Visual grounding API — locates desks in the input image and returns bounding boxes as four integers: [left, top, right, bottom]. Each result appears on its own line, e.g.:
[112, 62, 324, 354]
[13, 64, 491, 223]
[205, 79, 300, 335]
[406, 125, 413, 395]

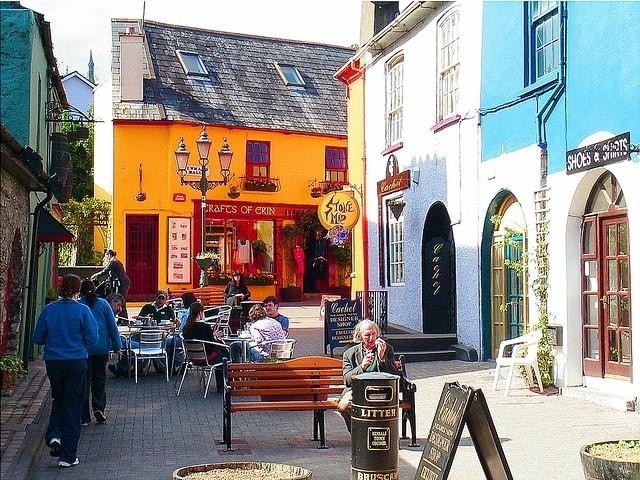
[129, 323, 176, 375]
[222, 336, 266, 362]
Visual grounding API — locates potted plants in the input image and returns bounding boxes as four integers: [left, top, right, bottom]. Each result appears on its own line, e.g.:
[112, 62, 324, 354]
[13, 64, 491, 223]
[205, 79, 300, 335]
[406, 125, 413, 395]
[580, 440, 640, 480]
[0, 354, 27, 392]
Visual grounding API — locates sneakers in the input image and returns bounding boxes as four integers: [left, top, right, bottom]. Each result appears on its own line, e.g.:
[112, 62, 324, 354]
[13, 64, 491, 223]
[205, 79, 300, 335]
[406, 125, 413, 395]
[108, 363, 121, 378]
[123, 368, 135, 377]
[59, 458, 79, 467]
[94, 410, 107, 424]
[50, 438, 61, 457]
[81, 423, 90, 427]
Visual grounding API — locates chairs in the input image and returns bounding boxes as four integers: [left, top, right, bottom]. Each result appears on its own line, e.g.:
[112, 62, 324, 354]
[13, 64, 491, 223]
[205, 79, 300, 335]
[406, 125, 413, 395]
[129, 329, 169, 383]
[200, 314, 224, 332]
[167, 298, 201, 311]
[493, 329, 544, 396]
[204, 305, 232, 335]
[246, 339, 296, 362]
[177, 339, 236, 399]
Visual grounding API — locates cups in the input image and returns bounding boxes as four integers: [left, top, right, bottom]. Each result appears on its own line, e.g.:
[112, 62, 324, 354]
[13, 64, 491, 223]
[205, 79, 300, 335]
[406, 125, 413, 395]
[240, 331, 248, 337]
[224, 327, 228, 337]
[160, 320, 169, 326]
[135, 320, 142, 326]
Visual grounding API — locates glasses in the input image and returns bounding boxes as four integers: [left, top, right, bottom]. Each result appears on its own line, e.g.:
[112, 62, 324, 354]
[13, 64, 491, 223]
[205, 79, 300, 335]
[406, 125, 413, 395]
[158, 298, 165, 300]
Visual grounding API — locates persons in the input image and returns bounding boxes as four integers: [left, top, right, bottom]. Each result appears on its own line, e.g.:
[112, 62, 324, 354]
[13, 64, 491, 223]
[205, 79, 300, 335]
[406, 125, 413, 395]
[82, 279, 123, 427]
[224, 271, 251, 302]
[263, 296, 290, 331]
[183, 302, 230, 394]
[166, 292, 198, 376]
[231, 303, 286, 362]
[32, 274, 99, 466]
[337, 320, 404, 435]
[138, 292, 175, 323]
[91, 249, 132, 325]
[105, 292, 139, 377]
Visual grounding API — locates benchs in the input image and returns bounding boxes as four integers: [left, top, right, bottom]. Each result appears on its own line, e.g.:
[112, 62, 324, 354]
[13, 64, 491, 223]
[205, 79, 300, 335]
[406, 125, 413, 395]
[222, 355, 420, 452]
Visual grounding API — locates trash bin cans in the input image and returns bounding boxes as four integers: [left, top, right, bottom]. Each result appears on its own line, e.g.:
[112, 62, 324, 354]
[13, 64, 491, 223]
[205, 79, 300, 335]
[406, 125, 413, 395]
[352, 372, 399, 480]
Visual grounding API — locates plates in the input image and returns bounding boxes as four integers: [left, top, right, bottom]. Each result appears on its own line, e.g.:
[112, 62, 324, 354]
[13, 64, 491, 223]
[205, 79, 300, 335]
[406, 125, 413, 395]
[159, 322, 174, 327]
[132, 323, 144, 325]
[239, 336, 251, 338]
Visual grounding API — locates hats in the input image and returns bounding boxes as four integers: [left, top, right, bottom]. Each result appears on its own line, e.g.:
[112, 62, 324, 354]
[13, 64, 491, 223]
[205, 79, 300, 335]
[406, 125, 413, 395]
[157, 290, 167, 298]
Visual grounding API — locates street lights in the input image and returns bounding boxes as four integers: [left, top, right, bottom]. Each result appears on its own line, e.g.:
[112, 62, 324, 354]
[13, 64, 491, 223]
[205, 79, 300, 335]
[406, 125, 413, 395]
[173, 122, 236, 288]
